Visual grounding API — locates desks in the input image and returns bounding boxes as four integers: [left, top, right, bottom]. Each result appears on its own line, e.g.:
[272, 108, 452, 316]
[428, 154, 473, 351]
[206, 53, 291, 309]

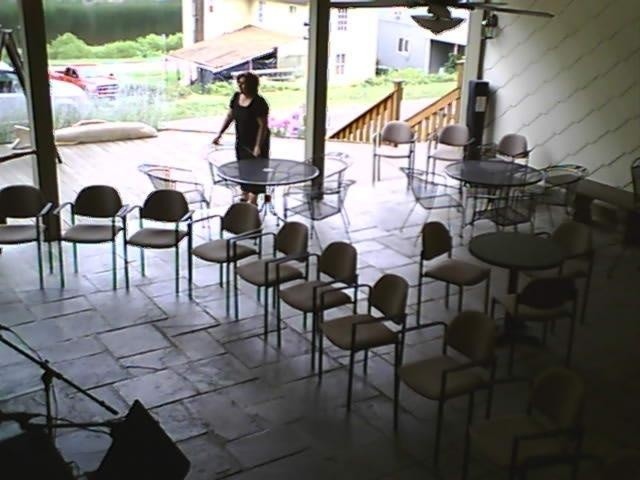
[216, 159, 320, 246]
[469, 232, 570, 347]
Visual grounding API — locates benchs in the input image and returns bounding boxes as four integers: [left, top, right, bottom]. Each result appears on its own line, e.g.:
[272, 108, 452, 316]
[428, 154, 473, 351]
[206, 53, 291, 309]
[546, 171, 639, 246]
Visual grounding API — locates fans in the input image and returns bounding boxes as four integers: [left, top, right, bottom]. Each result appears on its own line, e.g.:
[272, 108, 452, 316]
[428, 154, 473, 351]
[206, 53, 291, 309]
[328, 0, 559, 36]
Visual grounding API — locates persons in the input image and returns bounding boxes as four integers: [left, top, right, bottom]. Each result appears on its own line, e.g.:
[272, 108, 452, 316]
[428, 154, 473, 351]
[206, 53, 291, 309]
[212, 71, 272, 207]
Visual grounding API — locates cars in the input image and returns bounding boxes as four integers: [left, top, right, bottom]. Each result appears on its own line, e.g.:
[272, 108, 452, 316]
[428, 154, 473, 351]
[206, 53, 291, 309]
[0, 61, 89, 127]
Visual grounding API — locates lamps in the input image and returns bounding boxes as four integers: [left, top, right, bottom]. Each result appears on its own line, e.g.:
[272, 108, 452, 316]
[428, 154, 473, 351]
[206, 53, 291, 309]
[408, 3, 465, 36]
[479, 11, 501, 39]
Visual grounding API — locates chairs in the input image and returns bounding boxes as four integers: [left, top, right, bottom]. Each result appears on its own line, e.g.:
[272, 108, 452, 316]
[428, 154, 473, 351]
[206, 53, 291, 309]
[529, 220, 594, 344]
[486, 275, 580, 374]
[122, 188, 194, 297]
[1, 184, 54, 289]
[462, 366, 588, 477]
[372, 121, 417, 191]
[394, 310, 497, 461]
[605, 158, 639, 284]
[416, 221, 492, 324]
[203, 147, 276, 213]
[317, 273, 410, 414]
[275, 241, 359, 374]
[137, 164, 215, 241]
[398, 134, 589, 247]
[424, 124, 475, 192]
[53, 185, 130, 293]
[231, 222, 309, 346]
[190, 202, 263, 315]
[282, 151, 356, 255]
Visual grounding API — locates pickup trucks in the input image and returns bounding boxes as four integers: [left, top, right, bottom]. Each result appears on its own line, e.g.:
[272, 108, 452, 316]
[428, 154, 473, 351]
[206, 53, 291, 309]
[50, 63, 120, 101]
